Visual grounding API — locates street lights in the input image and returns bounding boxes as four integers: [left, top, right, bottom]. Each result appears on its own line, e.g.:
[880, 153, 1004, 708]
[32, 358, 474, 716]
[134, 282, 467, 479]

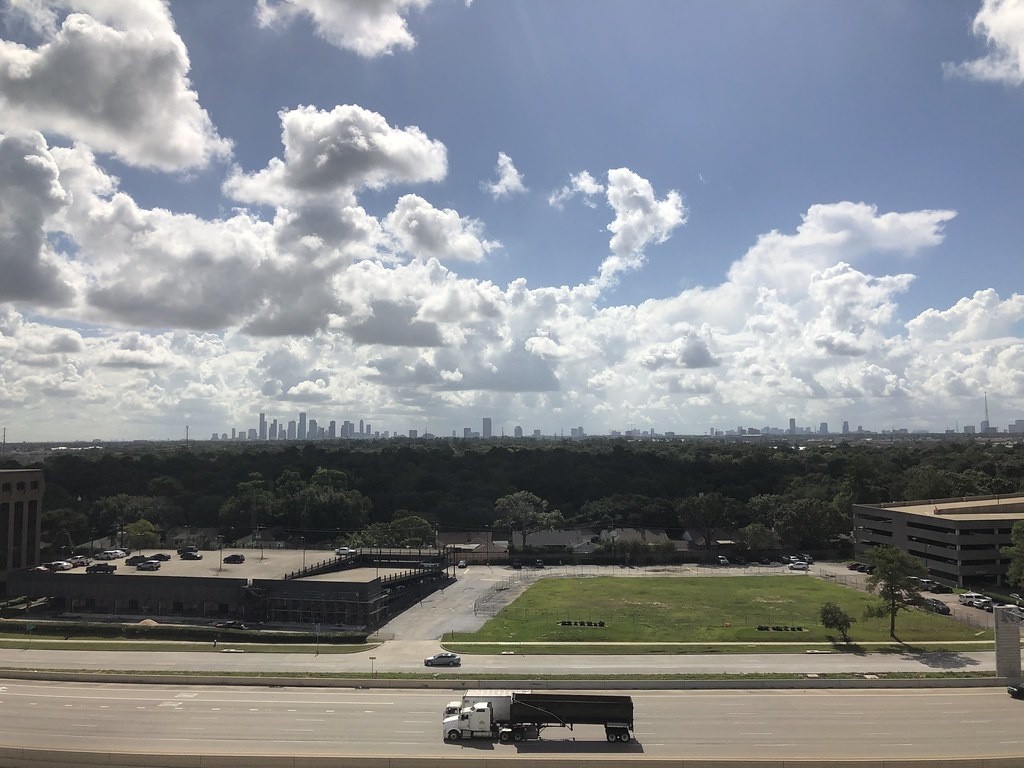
[185, 524, 191, 545]
[218, 534, 225, 570]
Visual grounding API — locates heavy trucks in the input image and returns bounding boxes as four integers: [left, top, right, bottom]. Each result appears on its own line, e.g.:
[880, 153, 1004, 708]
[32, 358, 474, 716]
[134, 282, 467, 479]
[442, 701, 540, 743]
[443, 689, 635, 741]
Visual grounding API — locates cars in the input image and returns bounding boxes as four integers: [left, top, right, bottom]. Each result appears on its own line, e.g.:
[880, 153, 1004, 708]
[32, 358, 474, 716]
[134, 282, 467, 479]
[1007, 681, 1024, 700]
[959, 593, 1005, 612]
[780, 554, 813, 570]
[717, 555, 729, 565]
[223, 554, 245, 563]
[29, 546, 203, 573]
[424, 653, 460, 666]
[216, 621, 249, 630]
[335, 547, 357, 555]
[847, 562, 953, 614]
[513, 558, 522, 568]
[458, 560, 468, 568]
[535, 559, 544, 568]
